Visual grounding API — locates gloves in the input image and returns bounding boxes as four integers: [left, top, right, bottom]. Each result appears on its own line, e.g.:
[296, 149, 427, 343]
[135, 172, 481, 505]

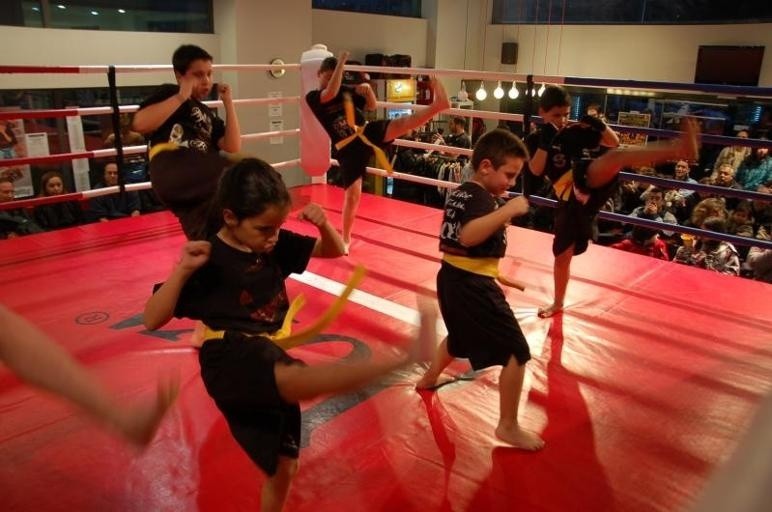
[577, 103, 608, 134]
[537, 122, 560, 151]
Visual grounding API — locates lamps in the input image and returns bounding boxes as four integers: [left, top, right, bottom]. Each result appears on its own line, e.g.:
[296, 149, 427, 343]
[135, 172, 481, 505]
[494, 0, 505, 100]
[477, 0, 486, 102]
[508, 0, 524, 99]
[538, 0, 553, 97]
[525, 0, 536, 97]
[457, 0, 471, 102]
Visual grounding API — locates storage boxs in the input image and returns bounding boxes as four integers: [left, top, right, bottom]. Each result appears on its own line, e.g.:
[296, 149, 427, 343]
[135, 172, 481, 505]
[365, 54, 411, 78]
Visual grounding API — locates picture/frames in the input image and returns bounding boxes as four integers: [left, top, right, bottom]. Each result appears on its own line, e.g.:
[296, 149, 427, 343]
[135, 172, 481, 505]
[617, 112, 651, 148]
[270, 58, 286, 78]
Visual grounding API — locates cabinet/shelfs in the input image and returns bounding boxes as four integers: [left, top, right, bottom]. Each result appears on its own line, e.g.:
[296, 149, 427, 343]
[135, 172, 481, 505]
[361, 78, 417, 195]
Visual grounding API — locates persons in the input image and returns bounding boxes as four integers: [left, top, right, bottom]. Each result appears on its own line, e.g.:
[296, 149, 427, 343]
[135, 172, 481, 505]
[597, 198, 625, 245]
[304, 50, 451, 255]
[417, 129, 546, 452]
[610, 221, 669, 261]
[671, 161, 700, 220]
[674, 217, 740, 276]
[745, 207, 772, 275]
[393, 130, 431, 201]
[618, 167, 644, 214]
[726, 201, 754, 239]
[0, 177, 43, 239]
[105, 114, 145, 159]
[744, 180, 772, 195]
[93, 163, 141, 220]
[677, 199, 724, 238]
[524, 84, 699, 318]
[432, 113, 470, 163]
[634, 167, 656, 195]
[736, 136, 772, 193]
[134, 45, 243, 243]
[1, 121, 17, 150]
[33, 172, 86, 232]
[145, 157, 438, 508]
[709, 162, 744, 191]
[621, 188, 678, 240]
[0, 303, 179, 453]
[711, 130, 751, 178]
